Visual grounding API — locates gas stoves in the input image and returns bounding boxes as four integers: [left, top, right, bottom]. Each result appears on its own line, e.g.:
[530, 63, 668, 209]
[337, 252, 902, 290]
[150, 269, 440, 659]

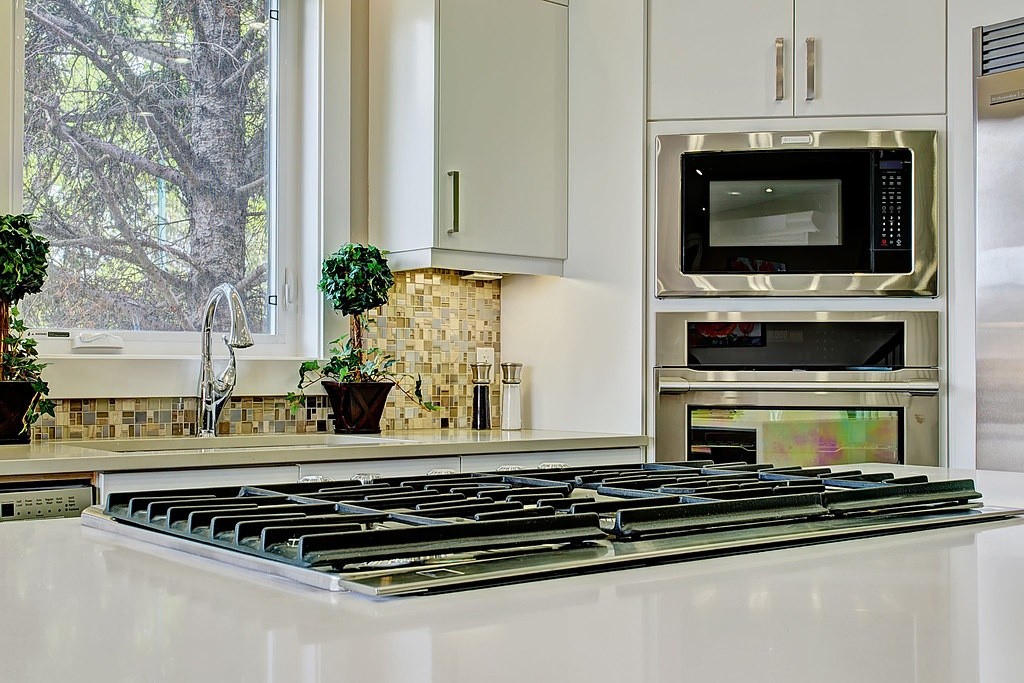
[81, 461, 1024, 595]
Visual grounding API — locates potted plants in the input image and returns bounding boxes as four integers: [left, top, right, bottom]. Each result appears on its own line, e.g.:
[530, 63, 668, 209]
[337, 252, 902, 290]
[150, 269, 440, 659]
[0, 212, 56, 445]
[282, 241, 441, 434]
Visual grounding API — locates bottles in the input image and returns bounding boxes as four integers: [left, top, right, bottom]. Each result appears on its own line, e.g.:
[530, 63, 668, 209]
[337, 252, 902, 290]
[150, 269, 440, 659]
[469, 363, 492, 430]
[501, 362, 522, 432]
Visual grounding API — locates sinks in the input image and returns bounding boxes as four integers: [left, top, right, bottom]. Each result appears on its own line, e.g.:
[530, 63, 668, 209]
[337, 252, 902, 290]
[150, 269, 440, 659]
[49, 434, 417, 452]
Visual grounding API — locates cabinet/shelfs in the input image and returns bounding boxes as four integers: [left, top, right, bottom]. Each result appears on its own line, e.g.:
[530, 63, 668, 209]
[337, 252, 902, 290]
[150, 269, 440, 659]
[369, 0, 571, 279]
[646, 0, 948, 121]
[99, 448, 641, 505]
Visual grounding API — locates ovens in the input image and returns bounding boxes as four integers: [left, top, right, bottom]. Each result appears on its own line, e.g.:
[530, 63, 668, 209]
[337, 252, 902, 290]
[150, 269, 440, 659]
[654, 312, 939, 466]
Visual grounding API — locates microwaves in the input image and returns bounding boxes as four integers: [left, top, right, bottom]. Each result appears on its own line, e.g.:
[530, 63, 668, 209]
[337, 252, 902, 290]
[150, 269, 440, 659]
[654, 130, 940, 298]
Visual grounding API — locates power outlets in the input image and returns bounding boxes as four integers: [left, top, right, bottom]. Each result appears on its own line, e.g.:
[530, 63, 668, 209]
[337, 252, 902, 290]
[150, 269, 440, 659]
[477, 346, 495, 385]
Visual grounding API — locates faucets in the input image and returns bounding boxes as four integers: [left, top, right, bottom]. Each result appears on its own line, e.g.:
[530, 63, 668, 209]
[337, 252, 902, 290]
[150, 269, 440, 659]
[198, 281, 255, 437]
